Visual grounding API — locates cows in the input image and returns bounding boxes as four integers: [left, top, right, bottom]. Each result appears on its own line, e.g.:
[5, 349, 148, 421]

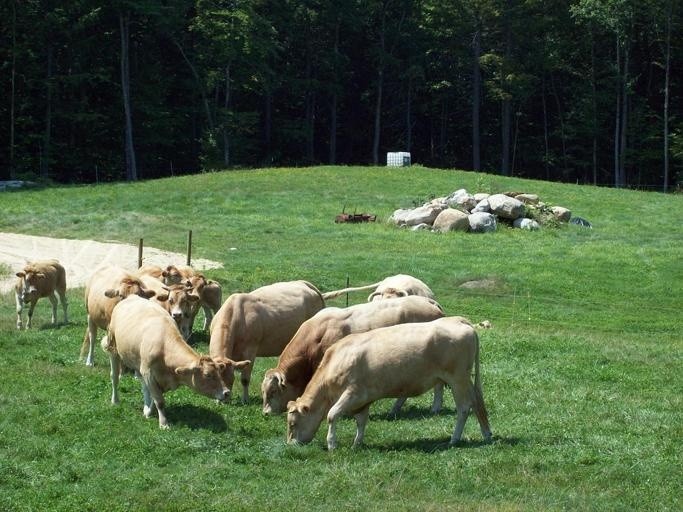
[205, 279, 326, 405]
[14, 260, 68, 331]
[260, 295, 446, 419]
[286, 316, 493, 453]
[105, 294, 231, 430]
[79, 263, 222, 379]
[321, 273, 435, 302]
